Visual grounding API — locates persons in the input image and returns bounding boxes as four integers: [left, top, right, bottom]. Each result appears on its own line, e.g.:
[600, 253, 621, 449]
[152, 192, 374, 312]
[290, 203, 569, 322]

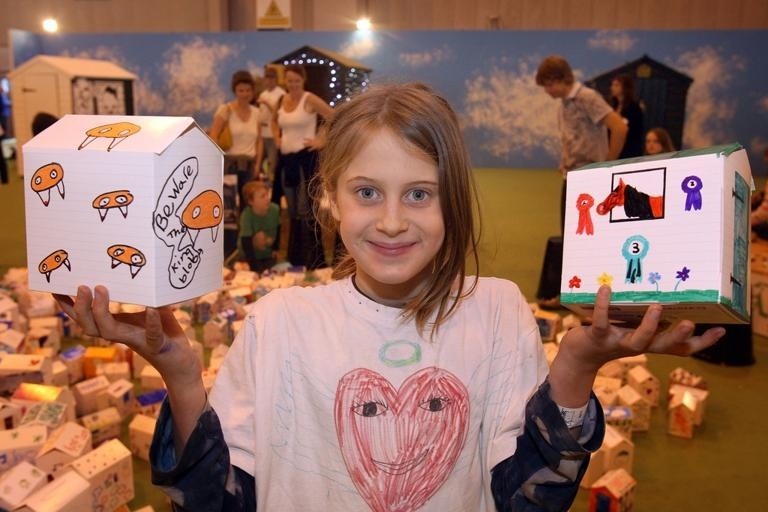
[48, 82, 726, 512]
[535, 57, 630, 312]
[210, 69, 264, 272]
[270, 62, 334, 271]
[643, 127, 676, 158]
[240, 182, 280, 273]
[255, 64, 286, 175]
[750, 180, 768, 246]
[601, 74, 647, 159]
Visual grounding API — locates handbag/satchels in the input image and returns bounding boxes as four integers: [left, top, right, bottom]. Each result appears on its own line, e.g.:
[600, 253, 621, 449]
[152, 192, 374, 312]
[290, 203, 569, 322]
[218, 103, 234, 152]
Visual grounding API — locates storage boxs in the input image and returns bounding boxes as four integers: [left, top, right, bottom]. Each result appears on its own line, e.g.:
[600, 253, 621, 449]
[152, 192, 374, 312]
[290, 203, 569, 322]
[19, 112, 230, 311]
[555, 137, 753, 330]
[3, 250, 746, 512]
